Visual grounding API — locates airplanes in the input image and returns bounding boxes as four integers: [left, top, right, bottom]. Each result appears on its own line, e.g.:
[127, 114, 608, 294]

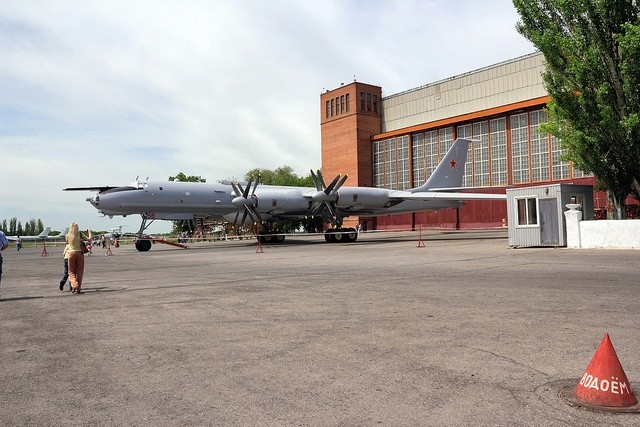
[5, 226, 69, 240]
[63, 137, 507, 252]
[92, 226, 122, 239]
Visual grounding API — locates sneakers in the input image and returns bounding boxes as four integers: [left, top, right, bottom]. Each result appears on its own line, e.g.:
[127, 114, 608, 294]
[75, 287, 80, 294]
[59, 281, 64, 290]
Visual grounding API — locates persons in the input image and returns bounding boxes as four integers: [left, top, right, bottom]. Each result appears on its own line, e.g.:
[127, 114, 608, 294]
[16, 235, 22, 250]
[101, 235, 106, 248]
[0, 231, 9, 283]
[59, 244, 72, 291]
[65, 223, 92, 294]
[176, 230, 236, 243]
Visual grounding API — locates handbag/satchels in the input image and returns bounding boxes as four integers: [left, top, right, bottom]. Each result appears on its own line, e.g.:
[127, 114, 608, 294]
[80, 233, 90, 254]
